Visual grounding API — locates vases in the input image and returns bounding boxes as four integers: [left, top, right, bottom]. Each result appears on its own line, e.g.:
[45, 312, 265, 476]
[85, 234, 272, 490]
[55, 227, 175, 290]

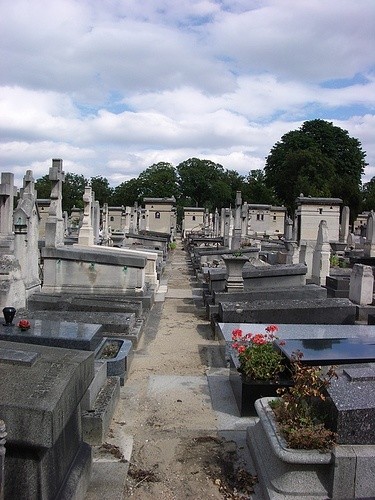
[229, 354, 296, 417]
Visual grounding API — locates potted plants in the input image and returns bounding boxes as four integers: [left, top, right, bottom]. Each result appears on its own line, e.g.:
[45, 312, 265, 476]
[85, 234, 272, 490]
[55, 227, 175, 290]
[245, 349, 340, 500]
[169, 243, 177, 253]
[93, 336, 134, 386]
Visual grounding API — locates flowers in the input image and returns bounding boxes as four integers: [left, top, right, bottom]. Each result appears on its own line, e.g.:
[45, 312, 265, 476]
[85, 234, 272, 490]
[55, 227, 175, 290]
[17, 320, 31, 332]
[232, 324, 286, 378]
